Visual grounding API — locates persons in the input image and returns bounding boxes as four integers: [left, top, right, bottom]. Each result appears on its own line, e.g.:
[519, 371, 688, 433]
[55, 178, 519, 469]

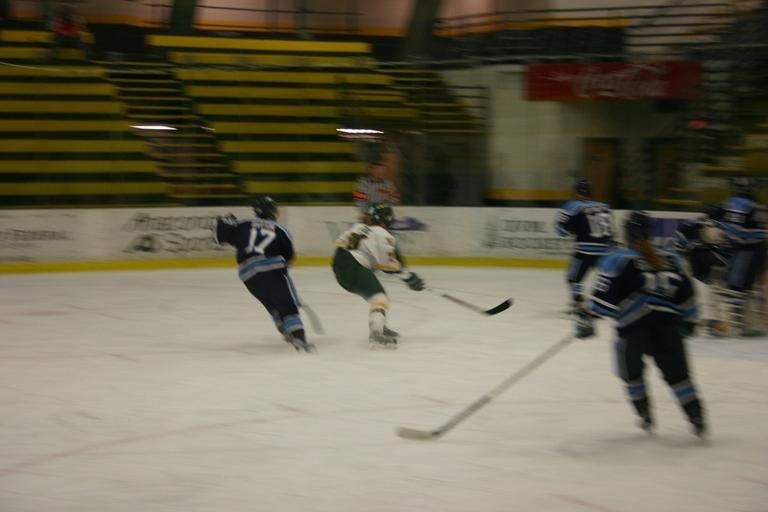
[353, 162, 400, 225]
[44, 4, 102, 66]
[554, 174, 768, 339]
[590, 209, 711, 437]
[330, 204, 423, 345]
[210, 195, 309, 352]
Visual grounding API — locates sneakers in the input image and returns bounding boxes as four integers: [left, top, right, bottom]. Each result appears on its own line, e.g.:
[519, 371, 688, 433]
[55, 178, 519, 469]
[368, 326, 401, 343]
[291, 337, 316, 351]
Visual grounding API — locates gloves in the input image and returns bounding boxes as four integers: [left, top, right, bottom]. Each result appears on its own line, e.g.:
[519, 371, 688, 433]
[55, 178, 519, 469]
[403, 272, 425, 291]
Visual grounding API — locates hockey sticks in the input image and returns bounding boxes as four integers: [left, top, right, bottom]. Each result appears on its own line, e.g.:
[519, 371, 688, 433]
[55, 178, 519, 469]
[419, 287, 513, 316]
[396, 334, 578, 439]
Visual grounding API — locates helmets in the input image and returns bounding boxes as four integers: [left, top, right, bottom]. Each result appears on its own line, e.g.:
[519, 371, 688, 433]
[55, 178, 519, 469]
[252, 194, 278, 220]
[367, 202, 395, 231]
[573, 178, 595, 197]
[625, 212, 651, 243]
